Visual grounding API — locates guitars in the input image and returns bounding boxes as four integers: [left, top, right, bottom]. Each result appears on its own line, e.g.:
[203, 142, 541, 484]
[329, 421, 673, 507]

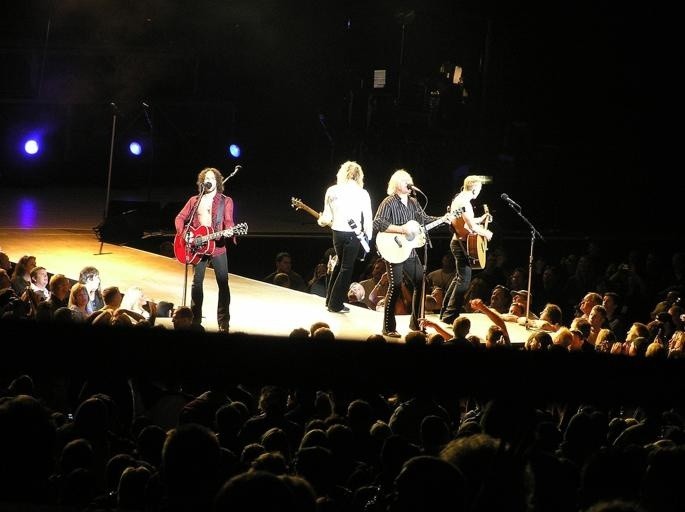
[173, 222, 248, 265]
[375, 207, 467, 265]
[467, 203, 490, 270]
[291, 197, 369, 262]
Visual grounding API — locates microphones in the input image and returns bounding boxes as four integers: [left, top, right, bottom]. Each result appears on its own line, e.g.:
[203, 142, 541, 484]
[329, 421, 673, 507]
[231, 165, 242, 177]
[407, 184, 421, 192]
[201, 182, 212, 188]
[501, 193, 517, 206]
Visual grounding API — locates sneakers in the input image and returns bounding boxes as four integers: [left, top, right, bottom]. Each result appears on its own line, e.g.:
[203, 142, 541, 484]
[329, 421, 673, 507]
[382, 330, 401, 338]
[329, 305, 350, 314]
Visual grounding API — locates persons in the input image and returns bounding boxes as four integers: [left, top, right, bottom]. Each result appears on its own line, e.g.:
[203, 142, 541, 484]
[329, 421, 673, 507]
[318, 162, 493, 338]
[2, 240, 685, 511]
[175, 169, 236, 334]
